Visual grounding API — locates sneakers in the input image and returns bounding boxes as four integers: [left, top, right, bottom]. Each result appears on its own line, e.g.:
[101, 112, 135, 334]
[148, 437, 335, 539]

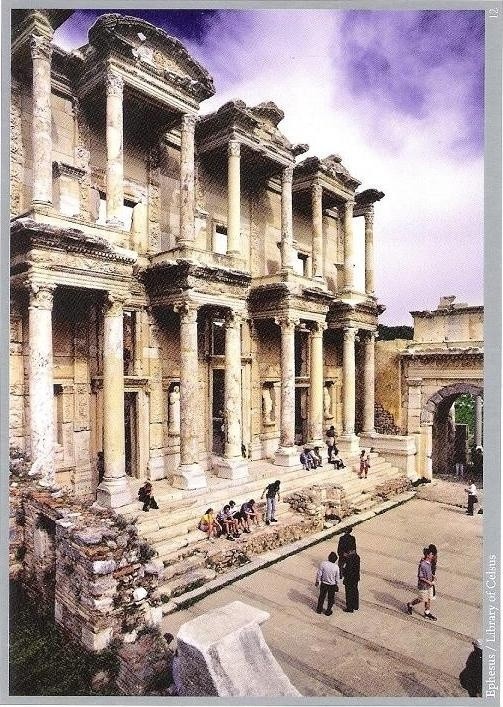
[266, 521, 270, 525]
[243, 528, 251, 533]
[406, 603, 412, 615]
[271, 519, 277, 521]
[424, 612, 437, 621]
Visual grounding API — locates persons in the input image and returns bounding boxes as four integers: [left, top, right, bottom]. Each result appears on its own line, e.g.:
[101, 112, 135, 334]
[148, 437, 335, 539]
[95, 450, 105, 483]
[138, 483, 159, 511]
[200, 479, 281, 543]
[337, 526, 357, 578]
[429, 543, 438, 600]
[461, 638, 483, 697]
[464, 479, 478, 516]
[299, 426, 370, 479]
[453, 435, 482, 476]
[344, 546, 361, 611]
[407, 550, 439, 622]
[315, 553, 340, 618]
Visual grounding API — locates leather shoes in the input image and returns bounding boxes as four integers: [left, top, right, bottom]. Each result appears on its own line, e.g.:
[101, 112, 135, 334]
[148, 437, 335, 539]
[345, 608, 353, 612]
